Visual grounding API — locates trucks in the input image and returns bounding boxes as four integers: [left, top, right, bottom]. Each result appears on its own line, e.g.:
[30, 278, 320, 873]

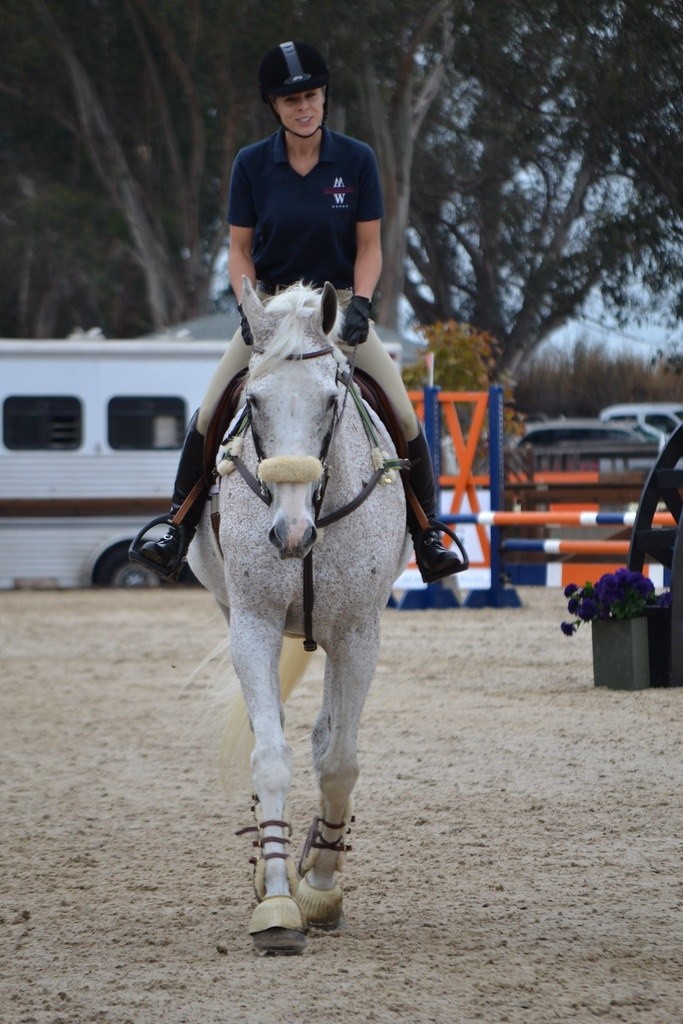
[0, 339, 227, 592]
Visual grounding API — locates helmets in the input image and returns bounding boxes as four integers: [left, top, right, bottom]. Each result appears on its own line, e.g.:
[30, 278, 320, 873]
[256, 41, 329, 107]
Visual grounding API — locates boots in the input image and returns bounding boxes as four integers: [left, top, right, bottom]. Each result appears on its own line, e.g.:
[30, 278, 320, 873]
[138, 407, 213, 582]
[400, 416, 462, 582]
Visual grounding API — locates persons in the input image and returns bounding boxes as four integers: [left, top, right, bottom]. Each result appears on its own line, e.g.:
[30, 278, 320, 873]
[140, 40, 461, 582]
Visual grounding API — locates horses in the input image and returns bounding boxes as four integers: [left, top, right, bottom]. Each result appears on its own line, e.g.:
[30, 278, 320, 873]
[184, 274, 410, 955]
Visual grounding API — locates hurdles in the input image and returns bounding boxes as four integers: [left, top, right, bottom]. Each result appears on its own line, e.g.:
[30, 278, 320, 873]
[397, 348, 679, 610]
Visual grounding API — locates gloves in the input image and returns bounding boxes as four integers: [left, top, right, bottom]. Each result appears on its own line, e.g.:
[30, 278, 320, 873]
[338, 295, 372, 346]
[236, 303, 254, 347]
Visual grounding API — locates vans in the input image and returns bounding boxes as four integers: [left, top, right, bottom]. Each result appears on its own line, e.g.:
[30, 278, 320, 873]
[599, 400, 683, 470]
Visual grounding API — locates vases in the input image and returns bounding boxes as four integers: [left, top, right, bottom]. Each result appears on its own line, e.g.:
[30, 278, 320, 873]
[592, 618, 650, 690]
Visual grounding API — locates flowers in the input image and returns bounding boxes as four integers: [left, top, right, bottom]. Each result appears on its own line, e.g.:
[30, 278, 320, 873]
[560, 566, 658, 636]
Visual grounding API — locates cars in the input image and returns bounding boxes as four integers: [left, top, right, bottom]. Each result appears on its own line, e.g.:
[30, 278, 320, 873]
[498, 416, 651, 473]
